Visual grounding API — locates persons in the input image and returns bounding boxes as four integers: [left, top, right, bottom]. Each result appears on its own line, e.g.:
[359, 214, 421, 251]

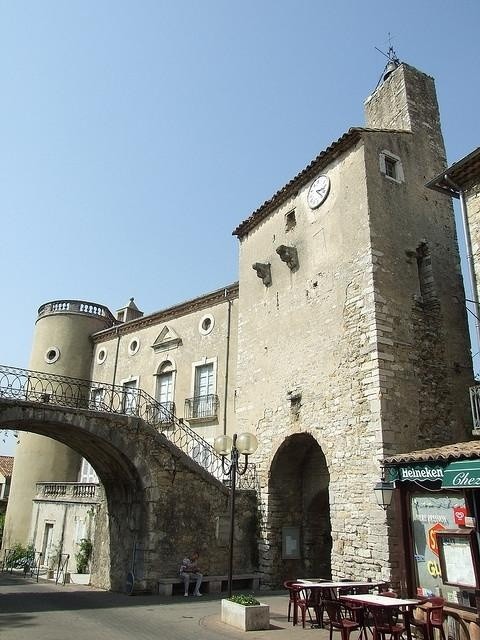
[179, 552, 204, 598]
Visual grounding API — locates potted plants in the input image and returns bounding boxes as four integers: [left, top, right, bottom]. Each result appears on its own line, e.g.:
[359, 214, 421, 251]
[220, 594, 270, 631]
[70, 538, 91, 585]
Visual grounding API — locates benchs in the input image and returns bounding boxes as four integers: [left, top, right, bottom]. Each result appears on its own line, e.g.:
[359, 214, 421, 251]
[158, 573, 260, 596]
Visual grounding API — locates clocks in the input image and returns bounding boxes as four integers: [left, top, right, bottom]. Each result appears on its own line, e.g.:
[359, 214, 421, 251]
[306, 175, 330, 209]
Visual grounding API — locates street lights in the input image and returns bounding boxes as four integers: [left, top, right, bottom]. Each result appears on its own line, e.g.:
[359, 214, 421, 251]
[213, 432, 258, 598]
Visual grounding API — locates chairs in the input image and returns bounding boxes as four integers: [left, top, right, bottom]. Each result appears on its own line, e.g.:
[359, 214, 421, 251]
[284, 579, 446, 640]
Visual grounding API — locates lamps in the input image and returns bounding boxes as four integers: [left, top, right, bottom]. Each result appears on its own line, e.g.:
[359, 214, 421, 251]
[373, 467, 397, 510]
